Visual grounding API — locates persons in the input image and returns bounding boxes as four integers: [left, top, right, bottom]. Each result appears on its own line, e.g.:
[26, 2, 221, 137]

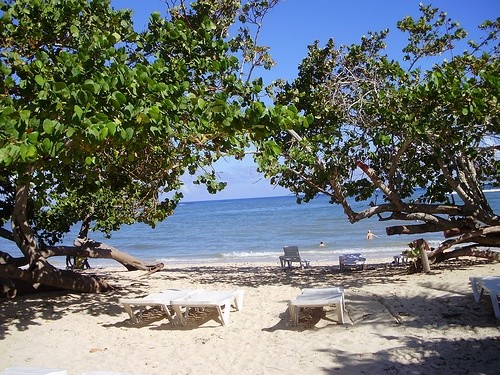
[66, 256, 92, 269]
[367, 230, 379, 239]
[320, 241, 324, 246]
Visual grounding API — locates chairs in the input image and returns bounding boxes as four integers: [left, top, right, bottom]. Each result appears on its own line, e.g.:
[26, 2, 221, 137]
[122, 288, 243, 330]
[339, 254, 366, 272]
[279, 246, 310, 270]
[286, 286, 344, 326]
[469, 276, 500, 319]
[393, 255, 413, 263]
